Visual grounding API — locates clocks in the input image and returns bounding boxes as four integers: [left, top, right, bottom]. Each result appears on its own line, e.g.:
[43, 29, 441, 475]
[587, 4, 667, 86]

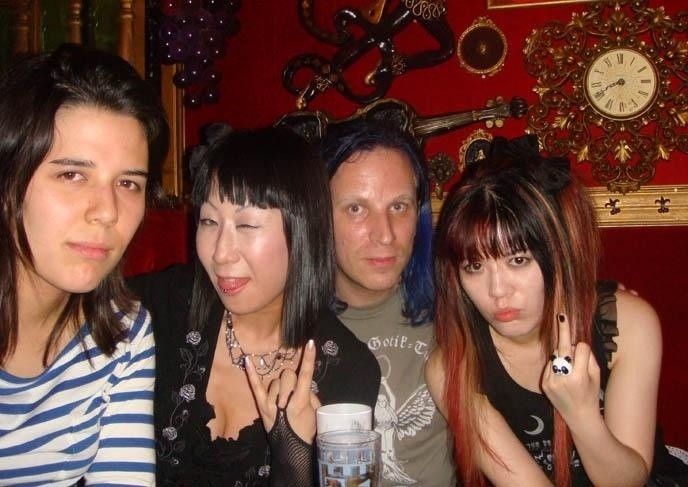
[523, 0, 688, 184]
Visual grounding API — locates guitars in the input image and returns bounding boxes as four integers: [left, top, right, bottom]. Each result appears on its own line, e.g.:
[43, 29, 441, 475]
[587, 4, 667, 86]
[272, 97, 525, 149]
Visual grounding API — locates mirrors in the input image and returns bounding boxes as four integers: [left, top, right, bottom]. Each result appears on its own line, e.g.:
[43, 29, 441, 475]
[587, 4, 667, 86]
[455, 15, 508, 75]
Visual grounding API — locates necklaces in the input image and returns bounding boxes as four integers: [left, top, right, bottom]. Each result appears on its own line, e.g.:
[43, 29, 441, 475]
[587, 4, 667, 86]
[223, 305, 299, 377]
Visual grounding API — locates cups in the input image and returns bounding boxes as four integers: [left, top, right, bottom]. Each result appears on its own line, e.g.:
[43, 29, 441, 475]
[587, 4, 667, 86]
[314, 403, 382, 487]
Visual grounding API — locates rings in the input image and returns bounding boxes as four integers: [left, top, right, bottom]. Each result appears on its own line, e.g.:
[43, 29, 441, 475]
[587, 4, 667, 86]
[548, 354, 576, 376]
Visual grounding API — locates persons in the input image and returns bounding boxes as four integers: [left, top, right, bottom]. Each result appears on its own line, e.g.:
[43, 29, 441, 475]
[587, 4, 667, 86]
[0, 44, 170, 487]
[422, 143, 686, 487]
[320, 116, 640, 486]
[374, 374, 399, 478]
[121, 121, 382, 487]
